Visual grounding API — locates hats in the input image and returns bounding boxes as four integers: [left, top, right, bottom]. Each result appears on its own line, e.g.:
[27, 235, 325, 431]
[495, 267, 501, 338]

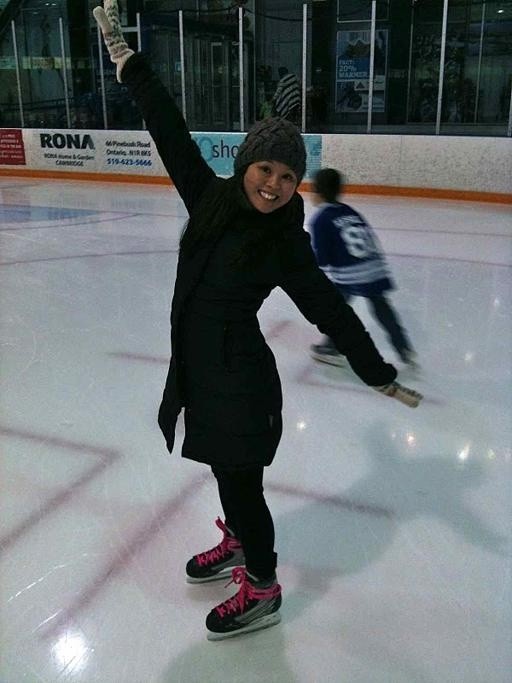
[315, 167, 340, 205]
[231, 115, 309, 186]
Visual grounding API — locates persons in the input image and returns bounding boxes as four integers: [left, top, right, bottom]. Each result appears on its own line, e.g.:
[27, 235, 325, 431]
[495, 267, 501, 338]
[303, 165, 417, 367]
[90, 0, 424, 636]
[270, 64, 303, 121]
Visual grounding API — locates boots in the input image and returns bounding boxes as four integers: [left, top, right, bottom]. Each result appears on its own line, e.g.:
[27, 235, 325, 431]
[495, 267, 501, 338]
[187, 524, 245, 579]
[206, 575, 283, 633]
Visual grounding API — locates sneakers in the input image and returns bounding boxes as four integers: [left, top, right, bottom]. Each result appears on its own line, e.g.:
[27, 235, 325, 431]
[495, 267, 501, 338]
[310, 338, 344, 356]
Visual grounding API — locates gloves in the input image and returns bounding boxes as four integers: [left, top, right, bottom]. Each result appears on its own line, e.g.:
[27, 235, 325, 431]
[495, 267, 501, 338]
[92, 0, 137, 85]
[371, 380, 424, 409]
